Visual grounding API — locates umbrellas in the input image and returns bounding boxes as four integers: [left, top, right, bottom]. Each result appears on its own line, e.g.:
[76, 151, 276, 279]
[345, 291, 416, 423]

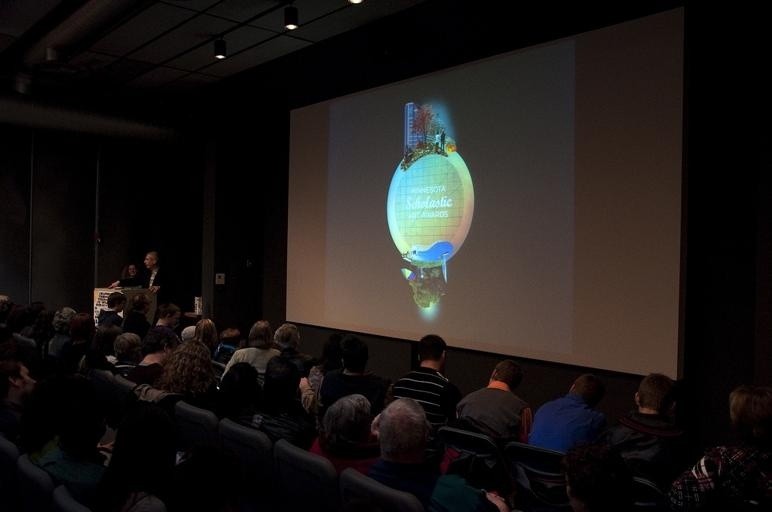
[400, 267, 417, 282]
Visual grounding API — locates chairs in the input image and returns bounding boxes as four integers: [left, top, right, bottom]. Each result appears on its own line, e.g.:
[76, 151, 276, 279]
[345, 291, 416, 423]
[0, 322, 772, 512]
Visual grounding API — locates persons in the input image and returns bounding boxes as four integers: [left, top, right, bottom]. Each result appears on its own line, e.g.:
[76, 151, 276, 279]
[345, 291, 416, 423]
[119, 262, 142, 279]
[440, 129, 446, 153]
[109, 251, 182, 306]
[435, 132, 441, 153]
[1, 292, 770, 510]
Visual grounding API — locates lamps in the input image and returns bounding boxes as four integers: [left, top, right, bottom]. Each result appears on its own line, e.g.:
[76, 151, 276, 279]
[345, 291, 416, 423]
[45, 48, 57, 64]
[16, 72, 32, 93]
[214, 35, 226, 59]
[284, 3, 298, 30]
